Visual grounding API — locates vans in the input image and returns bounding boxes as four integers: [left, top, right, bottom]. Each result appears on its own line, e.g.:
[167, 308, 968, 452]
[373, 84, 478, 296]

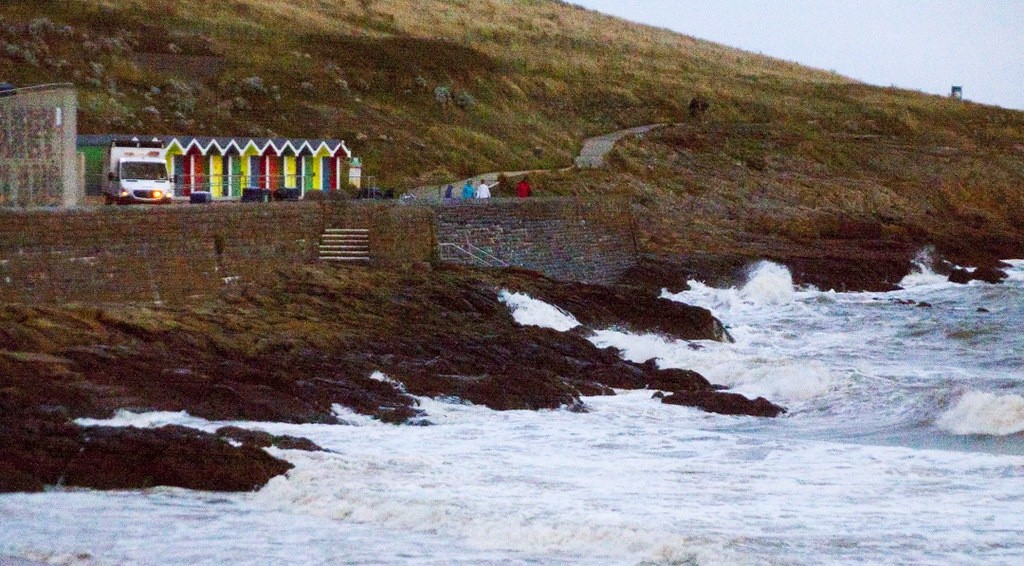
[100, 138, 173, 205]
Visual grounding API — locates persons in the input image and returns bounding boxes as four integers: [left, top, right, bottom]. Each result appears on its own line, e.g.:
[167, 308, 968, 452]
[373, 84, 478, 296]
[476, 179, 491, 198]
[515, 175, 533, 198]
[462, 179, 477, 199]
[444, 184, 457, 201]
